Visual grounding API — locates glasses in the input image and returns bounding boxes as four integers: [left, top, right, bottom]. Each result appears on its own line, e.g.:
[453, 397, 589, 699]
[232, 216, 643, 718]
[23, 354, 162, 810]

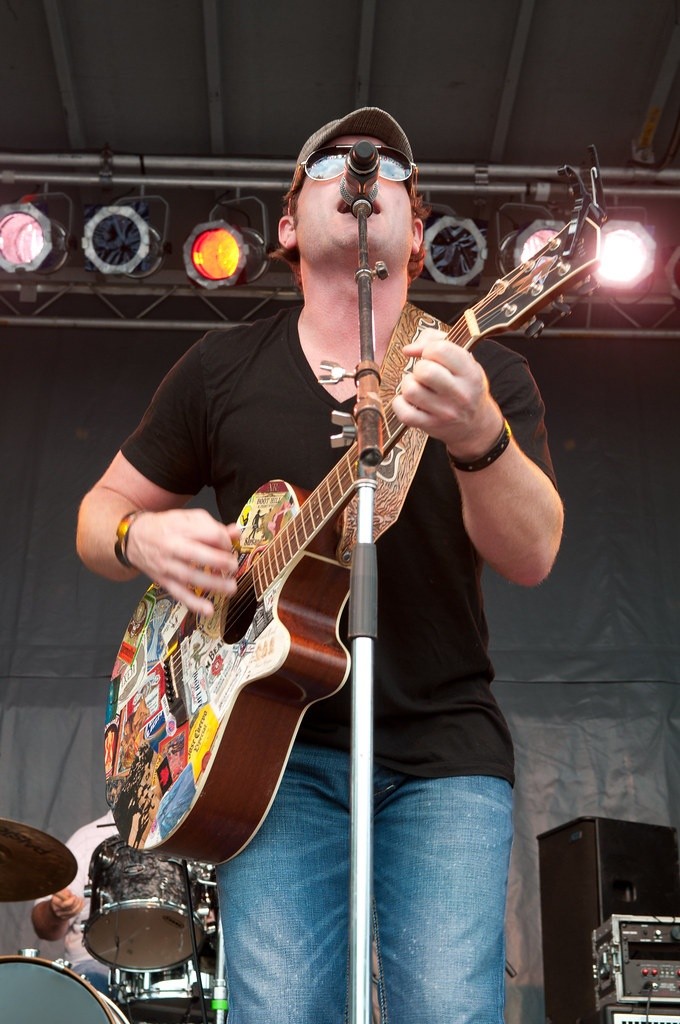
[288, 144, 418, 215]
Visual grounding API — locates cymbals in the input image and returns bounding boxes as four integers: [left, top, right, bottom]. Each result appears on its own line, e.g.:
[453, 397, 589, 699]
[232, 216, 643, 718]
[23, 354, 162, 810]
[0, 818, 79, 902]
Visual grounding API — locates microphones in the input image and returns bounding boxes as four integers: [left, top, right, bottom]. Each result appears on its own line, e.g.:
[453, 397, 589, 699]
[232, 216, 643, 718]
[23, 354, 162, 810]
[339, 141, 380, 205]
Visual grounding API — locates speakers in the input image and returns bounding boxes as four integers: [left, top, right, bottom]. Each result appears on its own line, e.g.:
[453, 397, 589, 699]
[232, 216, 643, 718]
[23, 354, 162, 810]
[537, 816, 680, 1024]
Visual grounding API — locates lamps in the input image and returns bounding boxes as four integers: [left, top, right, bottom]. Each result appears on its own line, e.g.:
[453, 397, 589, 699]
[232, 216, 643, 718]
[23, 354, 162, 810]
[0, 182, 680, 304]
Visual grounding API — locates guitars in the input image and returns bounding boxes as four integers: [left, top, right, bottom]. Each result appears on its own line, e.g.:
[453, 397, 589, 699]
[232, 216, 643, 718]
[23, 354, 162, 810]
[103, 214, 604, 865]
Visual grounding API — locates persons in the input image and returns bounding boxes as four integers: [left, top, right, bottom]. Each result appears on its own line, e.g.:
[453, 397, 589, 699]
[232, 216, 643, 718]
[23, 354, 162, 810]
[32, 808, 120, 997]
[71, 106, 568, 1024]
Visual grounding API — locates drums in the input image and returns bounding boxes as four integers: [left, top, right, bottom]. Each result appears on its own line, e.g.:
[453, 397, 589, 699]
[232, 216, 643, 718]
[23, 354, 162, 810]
[0, 949, 130, 1024]
[86, 832, 212, 974]
[107, 948, 220, 1024]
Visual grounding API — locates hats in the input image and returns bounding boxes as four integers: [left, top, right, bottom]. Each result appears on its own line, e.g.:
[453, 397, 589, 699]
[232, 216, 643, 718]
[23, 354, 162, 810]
[292, 107, 414, 190]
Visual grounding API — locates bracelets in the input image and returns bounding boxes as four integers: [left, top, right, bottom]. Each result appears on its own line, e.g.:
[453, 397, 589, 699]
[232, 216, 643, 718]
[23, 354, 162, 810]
[113, 509, 147, 569]
[446, 419, 512, 472]
